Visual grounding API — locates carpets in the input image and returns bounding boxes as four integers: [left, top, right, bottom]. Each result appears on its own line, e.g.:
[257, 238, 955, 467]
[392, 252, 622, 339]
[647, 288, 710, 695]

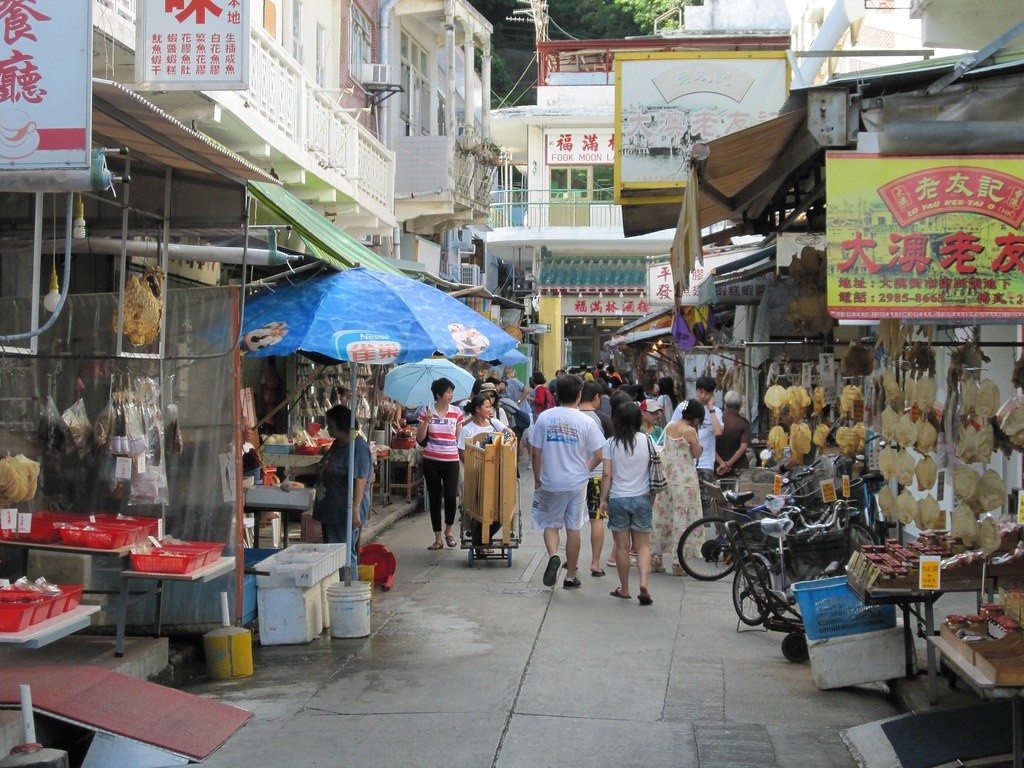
[0, 666, 254, 759]
[882, 702, 1014, 768]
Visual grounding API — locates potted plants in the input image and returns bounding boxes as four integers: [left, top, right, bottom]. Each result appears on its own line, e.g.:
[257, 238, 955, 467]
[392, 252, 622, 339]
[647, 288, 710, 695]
[463, 132, 504, 166]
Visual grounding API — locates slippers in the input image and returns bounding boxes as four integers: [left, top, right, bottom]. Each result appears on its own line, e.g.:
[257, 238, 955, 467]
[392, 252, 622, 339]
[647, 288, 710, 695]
[445, 534, 457, 548]
[606, 559, 618, 566]
[590, 568, 605, 576]
[563, 561, 578, 569]
[638, 590, 652, 603]
[428, 542, 444, 550]
[610, 587, 632, 598]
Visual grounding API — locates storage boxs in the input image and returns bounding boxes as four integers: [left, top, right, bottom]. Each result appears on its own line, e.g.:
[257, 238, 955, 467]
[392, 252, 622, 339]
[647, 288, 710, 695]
[0, 437, 346, 644]
[790, 575, 919, 690]
[0, 581, 84, 632]
[939, 621, 1024, 685]
[391, 438, 417, 449]
[739, 482, 782, 505]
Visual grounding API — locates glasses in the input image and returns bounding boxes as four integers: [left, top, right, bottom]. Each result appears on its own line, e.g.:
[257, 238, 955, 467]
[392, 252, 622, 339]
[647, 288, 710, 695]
[488, 395, 495, 398]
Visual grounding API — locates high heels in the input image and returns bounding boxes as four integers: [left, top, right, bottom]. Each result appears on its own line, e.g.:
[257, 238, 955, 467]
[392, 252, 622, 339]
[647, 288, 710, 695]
[672, 562, 688, 577]
[651, 556, 666, 573]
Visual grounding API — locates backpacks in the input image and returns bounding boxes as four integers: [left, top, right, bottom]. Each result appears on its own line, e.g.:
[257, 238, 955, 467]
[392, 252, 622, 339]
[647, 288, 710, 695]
[539, 385, 559, 412]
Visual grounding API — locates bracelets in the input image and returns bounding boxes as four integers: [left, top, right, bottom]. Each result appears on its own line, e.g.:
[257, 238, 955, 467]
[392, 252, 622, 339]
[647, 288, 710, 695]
[517, 400, 520, 403]
[709, 409, 715, 414]
[701, 446, 703, 452]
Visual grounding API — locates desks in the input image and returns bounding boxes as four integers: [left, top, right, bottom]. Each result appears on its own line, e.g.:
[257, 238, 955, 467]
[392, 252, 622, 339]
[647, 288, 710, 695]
[927, 636, 1024, 700]
[0, 606, 101, 650]
[845, 579, 1024, 706]
[0, 539, 236, 657]
[242, 448, 424, 549]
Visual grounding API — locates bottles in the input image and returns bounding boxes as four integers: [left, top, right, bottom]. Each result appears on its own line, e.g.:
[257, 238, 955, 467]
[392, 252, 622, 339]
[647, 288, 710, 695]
[945, 603, 1019, 642]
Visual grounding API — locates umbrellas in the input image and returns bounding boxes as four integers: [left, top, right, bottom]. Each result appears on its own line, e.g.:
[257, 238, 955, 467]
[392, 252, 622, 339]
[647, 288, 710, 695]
[383, 358, 475, 418]
[238, 260, 521, 587]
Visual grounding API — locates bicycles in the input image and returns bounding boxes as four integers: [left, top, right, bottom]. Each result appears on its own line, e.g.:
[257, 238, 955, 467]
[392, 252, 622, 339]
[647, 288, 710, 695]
[676, 435, 884, 633]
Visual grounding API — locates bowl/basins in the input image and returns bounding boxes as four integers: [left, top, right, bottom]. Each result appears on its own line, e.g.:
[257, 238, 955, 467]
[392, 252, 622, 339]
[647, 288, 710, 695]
[294, 446, 319, 455]
[392, 437, 414, 448]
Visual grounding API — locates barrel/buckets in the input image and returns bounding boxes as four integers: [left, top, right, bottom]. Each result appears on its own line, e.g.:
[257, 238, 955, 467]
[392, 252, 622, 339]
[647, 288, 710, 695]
[326, 579, 371, 638]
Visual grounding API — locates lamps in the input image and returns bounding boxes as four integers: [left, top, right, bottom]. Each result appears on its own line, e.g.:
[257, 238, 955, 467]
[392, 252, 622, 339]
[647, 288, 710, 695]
[599, 292, 603, 298]
[558, 292, 562, 298]
[578, 292, 582, 299]
[532, 161, 537, 175]
[619, 291, 623, 298]
[639, 292, 643, 297]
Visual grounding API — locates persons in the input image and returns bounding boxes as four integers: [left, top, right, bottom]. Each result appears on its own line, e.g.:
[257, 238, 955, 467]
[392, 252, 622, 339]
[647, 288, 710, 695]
[649, 398, 706, 576]
[288, 405, 373, 581]
[671, 376, 725, 527]
[394, 404, 427, 427]
[569, 360, 677, 443]
[562, 380, 613, 577]
[458, 358, 565, 553]
[415, 377, 465, 550]
[714, 391, 752, 490]
[599, 403, 659, 605]
[606, 391, 637, 566]
[230, 417, 260, 470]
[528, 375, 606, 590]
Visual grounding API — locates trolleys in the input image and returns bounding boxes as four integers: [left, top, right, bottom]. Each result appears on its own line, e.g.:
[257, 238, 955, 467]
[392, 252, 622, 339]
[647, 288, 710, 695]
[457, 478, 522, 567]
[724, 520, 809, 662]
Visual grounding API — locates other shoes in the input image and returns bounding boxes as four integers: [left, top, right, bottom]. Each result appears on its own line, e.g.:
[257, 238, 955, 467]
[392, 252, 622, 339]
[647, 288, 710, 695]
[629, 549, 637, 555]
[526, 460, 533, 469]
[563, 576, 582, 589]
[543, 555, 562, 585]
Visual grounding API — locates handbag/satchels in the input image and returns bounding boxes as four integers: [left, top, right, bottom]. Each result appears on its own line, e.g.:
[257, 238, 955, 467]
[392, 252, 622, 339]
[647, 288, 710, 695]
[645, 432, 668, 494]
[416, 406, 429, 448]
[513, 410, 531, 428]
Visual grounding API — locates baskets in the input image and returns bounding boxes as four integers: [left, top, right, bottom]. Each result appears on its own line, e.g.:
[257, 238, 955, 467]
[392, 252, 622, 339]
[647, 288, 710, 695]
[787, 510, 848, 576]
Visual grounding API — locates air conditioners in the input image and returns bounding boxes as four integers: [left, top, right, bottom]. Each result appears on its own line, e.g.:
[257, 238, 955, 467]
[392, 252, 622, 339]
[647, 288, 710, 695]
[460, 264, 480, 286]
[361, 64, 391, 90]
[454, 228, 471, 248]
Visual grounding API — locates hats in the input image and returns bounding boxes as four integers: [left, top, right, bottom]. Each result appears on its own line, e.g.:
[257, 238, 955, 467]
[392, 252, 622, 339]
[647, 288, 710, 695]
[640, 399, 663, 413]
[480, 383, 498, 395]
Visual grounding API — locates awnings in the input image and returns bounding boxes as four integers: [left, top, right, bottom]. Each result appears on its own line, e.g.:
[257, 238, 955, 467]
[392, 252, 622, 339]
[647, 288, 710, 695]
[249, 181, 405, 276]
[601, 107, 807, 369]
